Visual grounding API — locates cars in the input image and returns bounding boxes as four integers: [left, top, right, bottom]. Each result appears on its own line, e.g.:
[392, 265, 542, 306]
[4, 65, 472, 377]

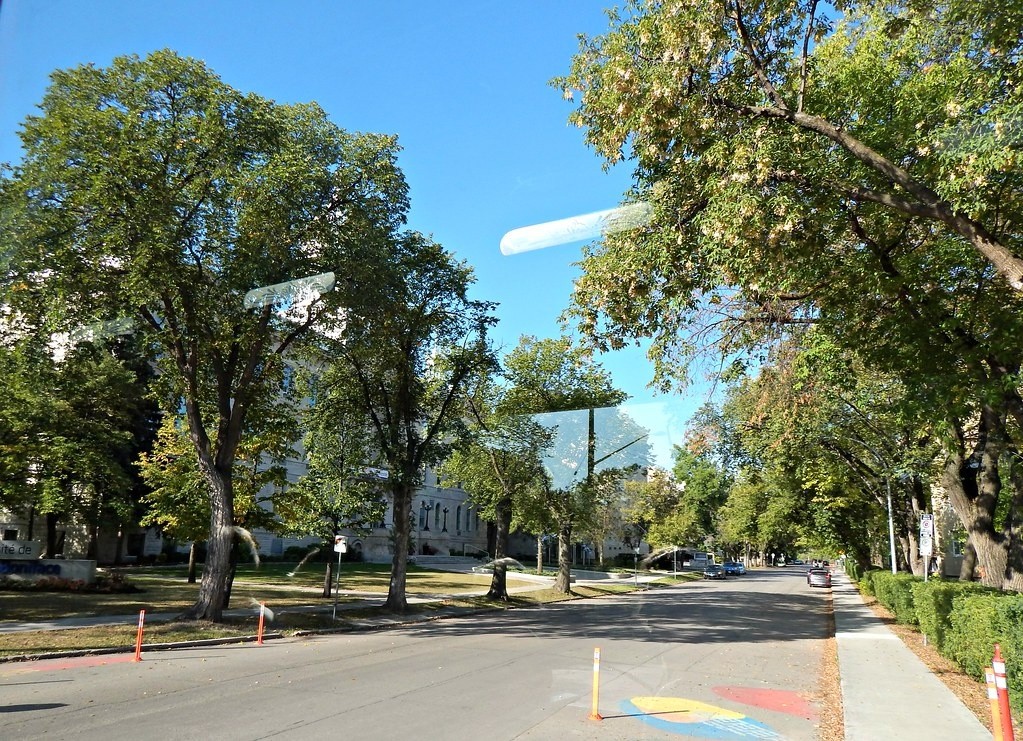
[703, 565, 726, 580]
[807, 570, 832, 587]
[807, 568, 824, 584]
[738, 563, 746, 575]
[723, 562, 740, 576]
[795, 560, 803, 564]
[813, 559, 823, 568]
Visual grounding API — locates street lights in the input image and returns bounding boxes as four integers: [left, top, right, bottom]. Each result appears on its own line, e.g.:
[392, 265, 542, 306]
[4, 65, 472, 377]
[823, 432, 896, 575]
